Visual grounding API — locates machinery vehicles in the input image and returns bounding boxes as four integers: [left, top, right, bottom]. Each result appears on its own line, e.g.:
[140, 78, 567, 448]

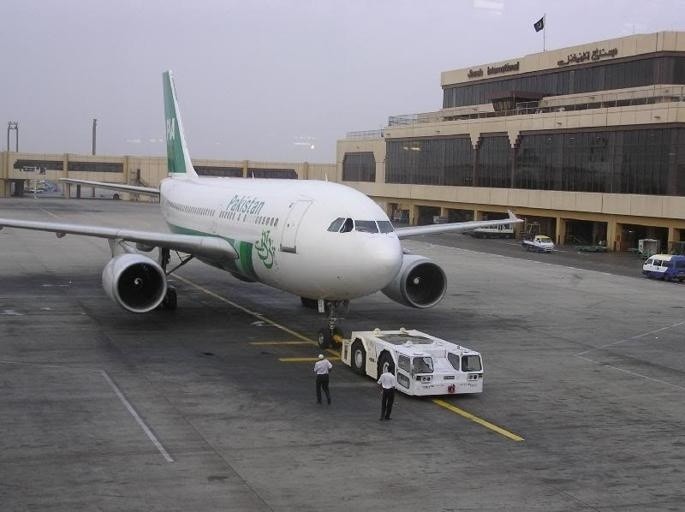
[340, 326, 487, 397]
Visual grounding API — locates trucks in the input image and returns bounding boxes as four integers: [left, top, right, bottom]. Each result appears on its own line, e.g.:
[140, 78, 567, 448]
[473, 223, 513, 240]
[522, 234, 556, 252]
[636, 239, 661, 259]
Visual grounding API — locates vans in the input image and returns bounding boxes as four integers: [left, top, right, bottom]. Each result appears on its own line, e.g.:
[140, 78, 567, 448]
[642, 254, 685, 283]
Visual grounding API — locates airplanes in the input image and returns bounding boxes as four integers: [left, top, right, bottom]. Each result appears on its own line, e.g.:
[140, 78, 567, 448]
[0, 70, 526, 349]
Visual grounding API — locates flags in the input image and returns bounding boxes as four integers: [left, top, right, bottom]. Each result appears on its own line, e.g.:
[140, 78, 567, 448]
[534, 17, 544, 32]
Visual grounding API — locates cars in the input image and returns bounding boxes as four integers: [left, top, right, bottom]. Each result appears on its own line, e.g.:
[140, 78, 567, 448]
[26, 179, 56, 194]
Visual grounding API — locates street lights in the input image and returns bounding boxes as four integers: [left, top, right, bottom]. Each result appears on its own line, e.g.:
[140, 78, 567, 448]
[6, 119, 19, 152]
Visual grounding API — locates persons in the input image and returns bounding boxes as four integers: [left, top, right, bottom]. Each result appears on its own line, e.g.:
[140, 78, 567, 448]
[375, 363, 397, 421]
[314, 352, 334, 405]
